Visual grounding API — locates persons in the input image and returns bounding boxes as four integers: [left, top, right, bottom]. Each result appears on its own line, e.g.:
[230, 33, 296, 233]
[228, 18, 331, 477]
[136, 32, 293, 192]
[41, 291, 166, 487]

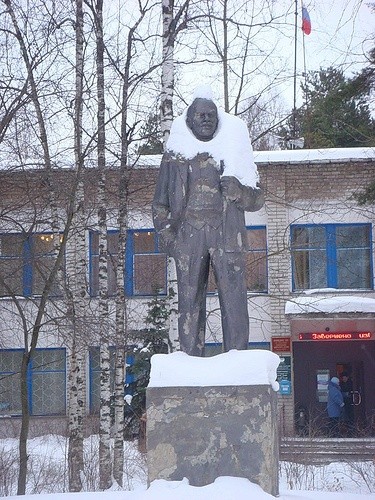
[326, 376, 345, 438]
[151, 97, 264, 358]
[338, 371, 354, 438]
